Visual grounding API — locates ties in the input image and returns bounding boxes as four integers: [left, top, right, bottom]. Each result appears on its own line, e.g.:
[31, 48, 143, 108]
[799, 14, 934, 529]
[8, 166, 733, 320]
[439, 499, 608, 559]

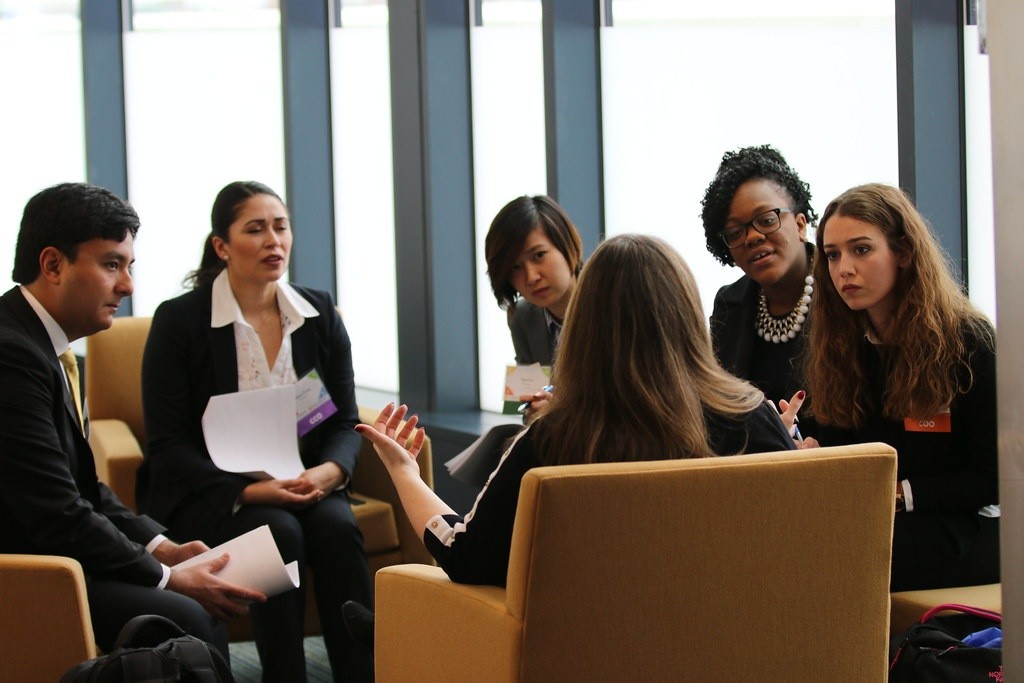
[57, 344, 87, 440]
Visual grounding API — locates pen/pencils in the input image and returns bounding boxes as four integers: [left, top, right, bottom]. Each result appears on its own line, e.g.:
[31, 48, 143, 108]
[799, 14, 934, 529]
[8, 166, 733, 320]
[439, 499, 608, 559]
[516, 383, 556, 415]
[792, 424, 808, 449]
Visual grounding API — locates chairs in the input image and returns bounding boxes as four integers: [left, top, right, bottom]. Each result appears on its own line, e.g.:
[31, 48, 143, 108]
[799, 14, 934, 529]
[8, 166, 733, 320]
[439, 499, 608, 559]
[83, 315, 435, 643]
[374, 443, 896, 683]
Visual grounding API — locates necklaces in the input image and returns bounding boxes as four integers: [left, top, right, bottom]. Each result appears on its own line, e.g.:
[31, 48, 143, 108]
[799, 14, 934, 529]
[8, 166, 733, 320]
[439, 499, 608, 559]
[754, 242, 819, 343]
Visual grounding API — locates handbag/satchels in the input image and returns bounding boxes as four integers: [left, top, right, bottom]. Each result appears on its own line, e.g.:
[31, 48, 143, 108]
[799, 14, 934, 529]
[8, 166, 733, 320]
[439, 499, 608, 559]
[889, 604, 1003, 683]
[58, 614, 237, 683]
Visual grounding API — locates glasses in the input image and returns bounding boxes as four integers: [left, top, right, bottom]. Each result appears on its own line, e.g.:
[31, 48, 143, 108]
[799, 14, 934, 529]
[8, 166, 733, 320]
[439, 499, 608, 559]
[721, 208, 797, 249]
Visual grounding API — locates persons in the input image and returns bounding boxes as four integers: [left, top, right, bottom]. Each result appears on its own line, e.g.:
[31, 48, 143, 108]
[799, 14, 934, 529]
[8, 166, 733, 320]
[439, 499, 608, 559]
[354, 233, 806, 589]
[0, 183, 268, 683]
[701, 147, 817, 423]
[486, 196, 584, 431]
[788, 183, 998, 590]
[133, 182, 375, 683]
